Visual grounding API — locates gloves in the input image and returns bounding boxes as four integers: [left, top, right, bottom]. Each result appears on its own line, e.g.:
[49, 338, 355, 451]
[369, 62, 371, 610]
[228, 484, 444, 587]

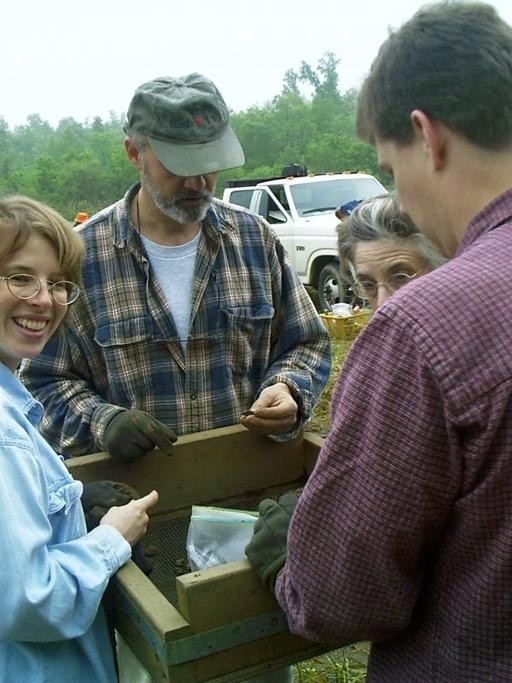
[101, 408, 180, 464]
[244, 491, 302, 592]
[81, 478, 154, 581]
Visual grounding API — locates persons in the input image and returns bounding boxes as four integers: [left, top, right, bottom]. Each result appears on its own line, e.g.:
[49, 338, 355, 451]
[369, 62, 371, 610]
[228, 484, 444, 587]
[0, 196, 163, 683]
[241, 0, 511, 681]
[337, 190, 452, 316]
[18, 72, 335, 683]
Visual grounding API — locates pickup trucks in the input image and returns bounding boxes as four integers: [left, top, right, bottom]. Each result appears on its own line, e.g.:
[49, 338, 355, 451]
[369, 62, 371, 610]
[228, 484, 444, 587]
[220, 172, 390, 316]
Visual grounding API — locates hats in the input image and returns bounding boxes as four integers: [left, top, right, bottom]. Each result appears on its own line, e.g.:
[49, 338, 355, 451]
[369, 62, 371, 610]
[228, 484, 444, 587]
[123, 70, 247, 179]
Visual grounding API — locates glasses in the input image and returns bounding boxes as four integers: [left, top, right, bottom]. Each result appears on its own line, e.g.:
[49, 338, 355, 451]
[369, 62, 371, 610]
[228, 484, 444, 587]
[0, 271, 85, 308]
[347, 262, 429, 300]
